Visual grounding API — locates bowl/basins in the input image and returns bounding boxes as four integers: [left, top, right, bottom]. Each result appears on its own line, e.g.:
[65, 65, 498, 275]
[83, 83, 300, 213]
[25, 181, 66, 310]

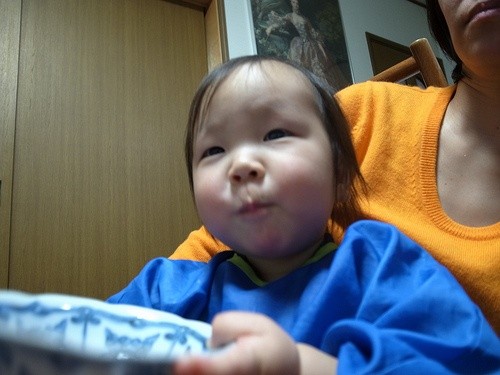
[0, 288, 236, 375]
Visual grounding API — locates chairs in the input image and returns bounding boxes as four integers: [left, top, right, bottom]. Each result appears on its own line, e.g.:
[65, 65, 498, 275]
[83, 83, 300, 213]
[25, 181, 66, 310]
[370, 38, 450, 88]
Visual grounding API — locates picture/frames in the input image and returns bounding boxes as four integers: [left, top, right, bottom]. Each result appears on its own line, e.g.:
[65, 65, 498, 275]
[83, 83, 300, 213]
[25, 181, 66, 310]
[246, 0, 356, 91]
